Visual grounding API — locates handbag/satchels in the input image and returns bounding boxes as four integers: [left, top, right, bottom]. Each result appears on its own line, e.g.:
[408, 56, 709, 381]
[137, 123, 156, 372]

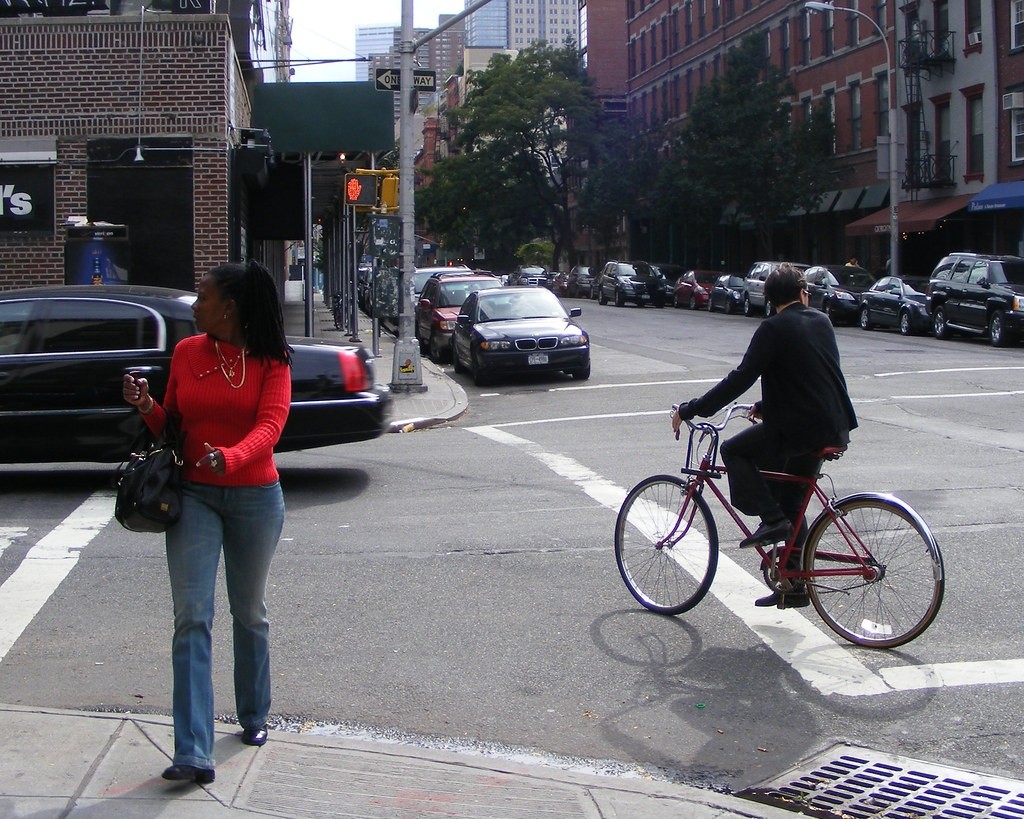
[112, 407, 187, 533]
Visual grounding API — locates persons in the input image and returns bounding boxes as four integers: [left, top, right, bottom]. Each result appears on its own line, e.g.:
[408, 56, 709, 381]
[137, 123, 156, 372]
[122, 261, 295, 783]
[845, 256, 859, 266]
[885, 254, 890, 274]
[672, 264, 859, 609]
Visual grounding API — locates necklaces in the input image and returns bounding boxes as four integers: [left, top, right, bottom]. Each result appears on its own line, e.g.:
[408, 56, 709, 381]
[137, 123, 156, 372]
[215, 337, 248, 388]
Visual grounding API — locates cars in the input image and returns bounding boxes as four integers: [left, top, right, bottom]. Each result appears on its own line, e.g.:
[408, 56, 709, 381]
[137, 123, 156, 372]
[858, 275, 932, 336]
[452, 286, 591, 387]
[548, 271, 570, 297]
[673, 269, 723, 311]
[474, 269, 515, 287]
[357, 266, 373, 317]
[707, 271, 745, 315]
[590, 271, 602, 300]
[1, 286, 394, 465]
[803, 265, 878, 327]
[648, 263, 690, 303]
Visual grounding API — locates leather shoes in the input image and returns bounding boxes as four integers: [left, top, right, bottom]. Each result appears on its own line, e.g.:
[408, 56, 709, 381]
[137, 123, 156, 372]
[242, 724, 268, 745]
[162, 765, 215, 783]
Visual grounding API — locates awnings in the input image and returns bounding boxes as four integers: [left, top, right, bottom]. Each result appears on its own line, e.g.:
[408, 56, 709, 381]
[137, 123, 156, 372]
[844, 191, 978, 236]
[788, 184, 889, 216]
[967, 179, 1024, 213]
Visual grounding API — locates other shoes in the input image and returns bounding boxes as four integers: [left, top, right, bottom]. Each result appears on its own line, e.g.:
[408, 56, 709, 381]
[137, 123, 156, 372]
[740, 519, 794, 548]
[755, 586, 810, 607]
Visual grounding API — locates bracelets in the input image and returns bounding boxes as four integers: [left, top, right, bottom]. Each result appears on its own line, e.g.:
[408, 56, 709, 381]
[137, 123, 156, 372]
[139, 395, 154, 414]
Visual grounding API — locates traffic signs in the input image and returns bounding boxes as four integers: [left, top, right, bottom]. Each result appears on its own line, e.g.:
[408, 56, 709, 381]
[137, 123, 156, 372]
[375, 67, 436, 92]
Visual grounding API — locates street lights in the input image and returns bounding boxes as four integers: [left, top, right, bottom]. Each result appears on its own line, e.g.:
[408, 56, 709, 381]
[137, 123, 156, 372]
[804, 2, 900, 278]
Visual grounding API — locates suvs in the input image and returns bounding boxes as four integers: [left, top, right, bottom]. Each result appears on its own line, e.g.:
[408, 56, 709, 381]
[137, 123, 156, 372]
[597, 258, 665, 309]
[508, 264, 554, 292]
[413, 263, 476, 306]
[414, 269, 512, 364]
[566, 265, 591, 298]
[742, 261, 811, 318]
[924, 252, 1024, 348]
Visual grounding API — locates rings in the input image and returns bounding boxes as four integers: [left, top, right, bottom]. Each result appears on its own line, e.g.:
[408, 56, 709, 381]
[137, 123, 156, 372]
[210, 453, 214, 460]
[211, 460, 217, 467]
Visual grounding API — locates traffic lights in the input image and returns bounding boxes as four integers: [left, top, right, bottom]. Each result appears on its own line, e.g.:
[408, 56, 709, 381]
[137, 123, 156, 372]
[343, 173, 378, 207]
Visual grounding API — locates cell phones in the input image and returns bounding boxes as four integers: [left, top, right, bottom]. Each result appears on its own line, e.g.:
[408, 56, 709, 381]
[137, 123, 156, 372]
[131, 370, 142, 401]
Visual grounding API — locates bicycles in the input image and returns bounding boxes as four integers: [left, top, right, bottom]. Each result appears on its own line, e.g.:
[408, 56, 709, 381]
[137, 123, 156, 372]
[615, 403, 944, 649]
